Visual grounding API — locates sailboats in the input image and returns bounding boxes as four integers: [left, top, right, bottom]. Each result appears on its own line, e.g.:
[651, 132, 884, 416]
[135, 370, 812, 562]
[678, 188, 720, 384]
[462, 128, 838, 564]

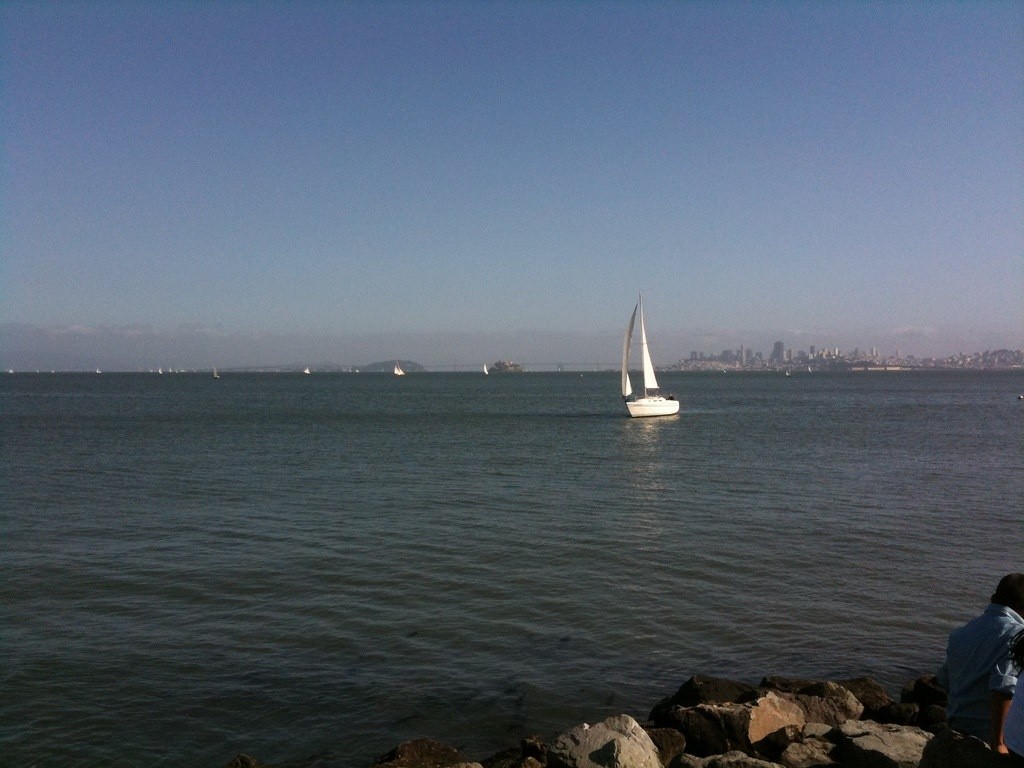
[394, 360, 406, 376]
[619, 292, 680, 417]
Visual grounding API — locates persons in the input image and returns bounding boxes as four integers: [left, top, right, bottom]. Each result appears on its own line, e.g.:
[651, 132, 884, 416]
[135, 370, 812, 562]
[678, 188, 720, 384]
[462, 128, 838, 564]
[667, 392, 676, 401]
[1007, 627, 1024, 766]
[934, 572, 1024, 755]
